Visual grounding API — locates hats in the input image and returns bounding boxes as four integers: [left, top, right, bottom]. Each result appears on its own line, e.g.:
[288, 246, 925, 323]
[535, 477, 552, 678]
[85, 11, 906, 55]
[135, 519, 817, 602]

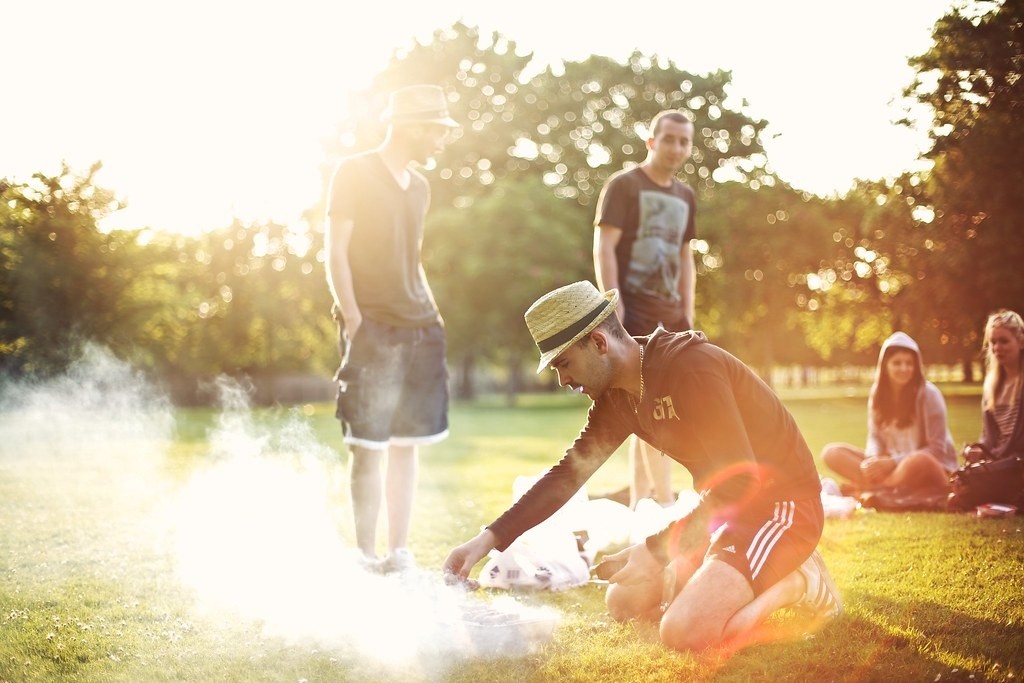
[524, 279, 620, 375]
[382, 84, 460, 127]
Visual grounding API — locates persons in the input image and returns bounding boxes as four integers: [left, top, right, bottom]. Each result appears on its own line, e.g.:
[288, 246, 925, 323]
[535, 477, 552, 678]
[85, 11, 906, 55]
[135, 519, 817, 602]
[943, 307, 1023, 514]
[318, 81, 453, 586]
[818, 329, 960, 504]
[440, 278, 843, 654]
[589, 110, 700, 525]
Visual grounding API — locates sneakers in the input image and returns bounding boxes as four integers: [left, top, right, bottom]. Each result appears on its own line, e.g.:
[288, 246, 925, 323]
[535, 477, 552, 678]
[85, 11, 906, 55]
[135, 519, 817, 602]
[783, 549, 843, 618]
